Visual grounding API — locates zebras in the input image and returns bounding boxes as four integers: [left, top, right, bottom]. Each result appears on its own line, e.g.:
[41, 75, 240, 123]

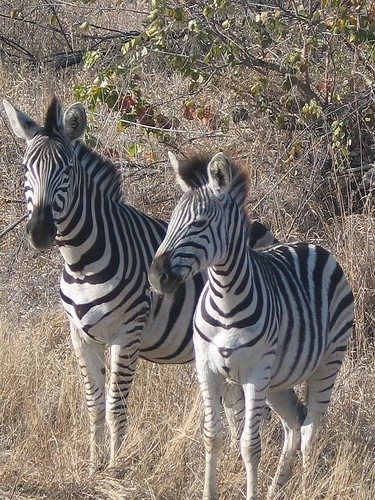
[3, 95, 277, 478]
[146, 152, 355, 500]
